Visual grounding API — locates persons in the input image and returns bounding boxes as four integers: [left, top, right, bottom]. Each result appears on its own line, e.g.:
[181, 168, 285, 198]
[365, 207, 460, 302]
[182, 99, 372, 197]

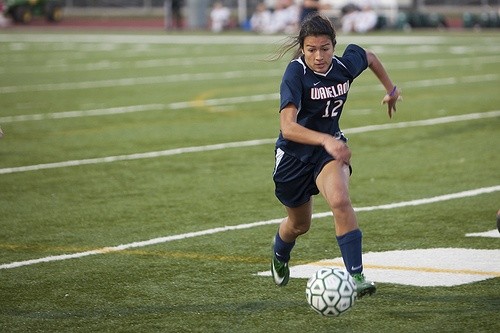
[270, 16, 399, 296]
[210, 1, 318, 33]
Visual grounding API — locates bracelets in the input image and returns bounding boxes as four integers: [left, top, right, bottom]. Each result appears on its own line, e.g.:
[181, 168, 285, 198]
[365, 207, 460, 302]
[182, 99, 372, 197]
[387, 85, 396, 96]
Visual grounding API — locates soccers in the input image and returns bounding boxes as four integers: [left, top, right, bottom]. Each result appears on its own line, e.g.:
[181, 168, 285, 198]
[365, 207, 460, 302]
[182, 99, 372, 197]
[305, 267, 357, 317]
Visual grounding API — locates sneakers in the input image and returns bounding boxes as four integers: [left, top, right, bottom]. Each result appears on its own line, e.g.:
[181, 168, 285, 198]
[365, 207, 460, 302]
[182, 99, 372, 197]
[351, 273, 377, 298]
[271, 236, 290, 286]
